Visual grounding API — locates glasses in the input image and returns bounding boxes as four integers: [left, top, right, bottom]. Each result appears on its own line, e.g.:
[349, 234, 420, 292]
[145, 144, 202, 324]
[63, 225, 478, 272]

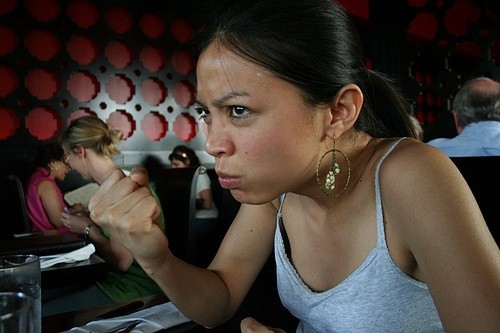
[63, 152, 71, 163]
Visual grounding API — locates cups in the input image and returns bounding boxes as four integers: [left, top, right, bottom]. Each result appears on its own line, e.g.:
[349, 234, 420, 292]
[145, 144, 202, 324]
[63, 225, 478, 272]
[0, 254, 42, 333]
[0, 292, 34, 333]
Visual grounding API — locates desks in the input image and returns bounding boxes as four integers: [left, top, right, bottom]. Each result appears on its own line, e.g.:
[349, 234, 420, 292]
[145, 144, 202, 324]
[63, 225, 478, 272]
[61, 291, 257, 333]
[0, 242, 110, 284]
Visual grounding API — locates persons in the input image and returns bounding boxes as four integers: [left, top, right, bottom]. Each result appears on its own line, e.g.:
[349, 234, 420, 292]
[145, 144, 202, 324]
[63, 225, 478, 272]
[169, 145, 219, 231]
[26, 141, 84, 235]
[89, 0, 500, 333]
[42, 116, 163, 316]
[423, 77, 500, 157]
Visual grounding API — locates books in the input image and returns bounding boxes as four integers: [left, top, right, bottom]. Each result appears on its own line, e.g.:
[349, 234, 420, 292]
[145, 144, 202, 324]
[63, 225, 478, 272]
[64, 183, 100, 207]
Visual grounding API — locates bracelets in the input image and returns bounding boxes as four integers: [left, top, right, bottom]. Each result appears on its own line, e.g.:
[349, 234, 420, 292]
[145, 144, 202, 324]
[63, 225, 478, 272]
[84, 223, 93, 235]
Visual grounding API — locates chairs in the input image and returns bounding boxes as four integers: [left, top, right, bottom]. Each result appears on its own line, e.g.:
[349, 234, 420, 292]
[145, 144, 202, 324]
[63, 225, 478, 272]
[149, 165, 242, 269]
[0, 174, 43, 239]
